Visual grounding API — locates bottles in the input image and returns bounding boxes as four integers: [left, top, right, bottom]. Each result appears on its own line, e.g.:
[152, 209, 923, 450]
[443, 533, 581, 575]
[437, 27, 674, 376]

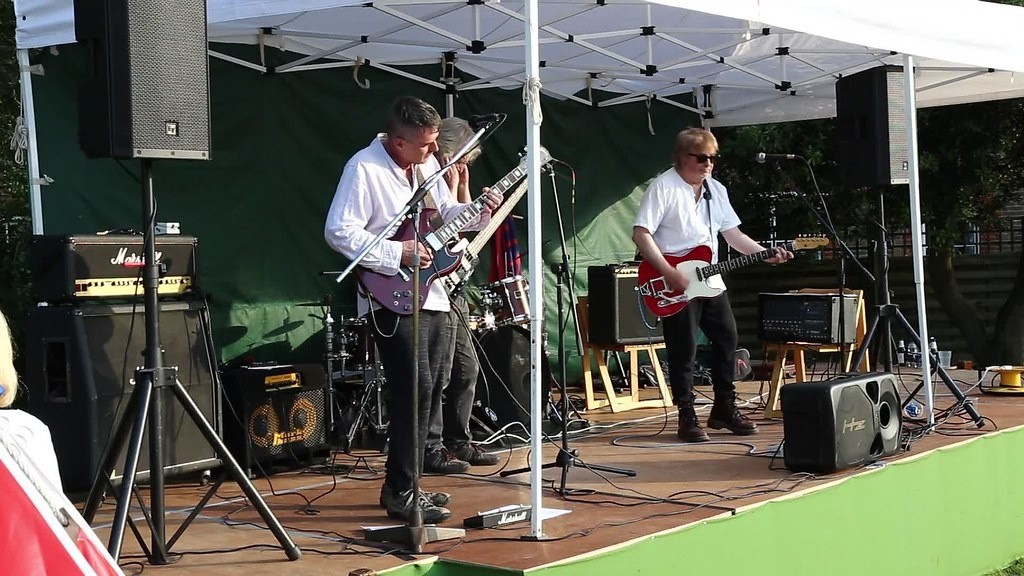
[929, 337, 939, 369]
[906, 341, 918, 369]
[897, 337, 906, 366]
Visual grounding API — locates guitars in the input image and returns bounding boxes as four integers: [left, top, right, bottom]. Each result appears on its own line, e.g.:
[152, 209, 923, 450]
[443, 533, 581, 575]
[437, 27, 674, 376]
[356, 145, 549, 315]
[636, 234, 829, 317]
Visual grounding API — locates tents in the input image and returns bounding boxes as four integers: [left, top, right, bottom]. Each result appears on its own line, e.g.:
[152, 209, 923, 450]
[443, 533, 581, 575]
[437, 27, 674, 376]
[2, 0, 1024, 539]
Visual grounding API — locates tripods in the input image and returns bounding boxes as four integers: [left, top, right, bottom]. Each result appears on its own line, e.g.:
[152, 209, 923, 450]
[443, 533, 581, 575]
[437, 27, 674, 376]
[344, 309, 390, 455]
[500, 158, 636, 498]
[847, 193, 985, 428]
[79, 156, 305, 570]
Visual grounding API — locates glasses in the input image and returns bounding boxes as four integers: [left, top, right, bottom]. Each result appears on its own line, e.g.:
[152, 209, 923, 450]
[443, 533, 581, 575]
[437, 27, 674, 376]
[685, 153, 720, 164]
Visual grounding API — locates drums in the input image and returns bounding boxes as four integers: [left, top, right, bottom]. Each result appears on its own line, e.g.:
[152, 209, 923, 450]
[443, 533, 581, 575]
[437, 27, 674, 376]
[480, 274, 536, 325]
[476, 327, 551, 433]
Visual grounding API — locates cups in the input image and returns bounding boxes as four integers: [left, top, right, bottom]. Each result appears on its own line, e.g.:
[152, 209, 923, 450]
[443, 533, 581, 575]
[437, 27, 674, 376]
[938, 351, 951, 369]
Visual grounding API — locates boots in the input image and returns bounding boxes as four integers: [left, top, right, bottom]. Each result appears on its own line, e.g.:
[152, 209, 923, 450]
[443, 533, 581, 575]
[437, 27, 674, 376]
[677, 404, 710, 442]
[707, 393, 758, 434]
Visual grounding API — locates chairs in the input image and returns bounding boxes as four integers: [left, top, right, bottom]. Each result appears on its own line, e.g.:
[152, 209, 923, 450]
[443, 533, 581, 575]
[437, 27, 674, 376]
[575, 296, 672, 413]
[765, 288, 869, 419]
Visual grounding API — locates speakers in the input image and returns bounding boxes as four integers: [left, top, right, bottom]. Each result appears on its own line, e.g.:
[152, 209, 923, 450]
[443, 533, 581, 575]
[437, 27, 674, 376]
[71, 0, 209, 160]
[586, 264, 666, 345]
[217, 362, 329, 481]
[30, 295, 223, 494]
[834, 65, 907, 194]
[779, 372, 904, 475]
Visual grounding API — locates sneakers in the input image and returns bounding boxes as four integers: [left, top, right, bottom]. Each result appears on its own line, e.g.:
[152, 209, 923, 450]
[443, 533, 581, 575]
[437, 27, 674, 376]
[387, 489, 451, 521]
[455, 442, 501, 464]
[380, 492, 450, 508]
[424, 447, 471, 473]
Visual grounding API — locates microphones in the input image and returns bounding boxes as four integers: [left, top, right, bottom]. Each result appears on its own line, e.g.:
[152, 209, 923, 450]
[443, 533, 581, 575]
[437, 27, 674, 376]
[468, 113, 500, 129]
[755, 151, 798, 164]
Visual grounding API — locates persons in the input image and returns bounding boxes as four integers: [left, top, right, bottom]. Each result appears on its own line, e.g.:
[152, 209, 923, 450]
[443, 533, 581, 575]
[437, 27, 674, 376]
[324, 95, 505, 523]
[0, 306, 64, 498]
[421, 117, 501, 475]
[632, 127, 794, 443]
